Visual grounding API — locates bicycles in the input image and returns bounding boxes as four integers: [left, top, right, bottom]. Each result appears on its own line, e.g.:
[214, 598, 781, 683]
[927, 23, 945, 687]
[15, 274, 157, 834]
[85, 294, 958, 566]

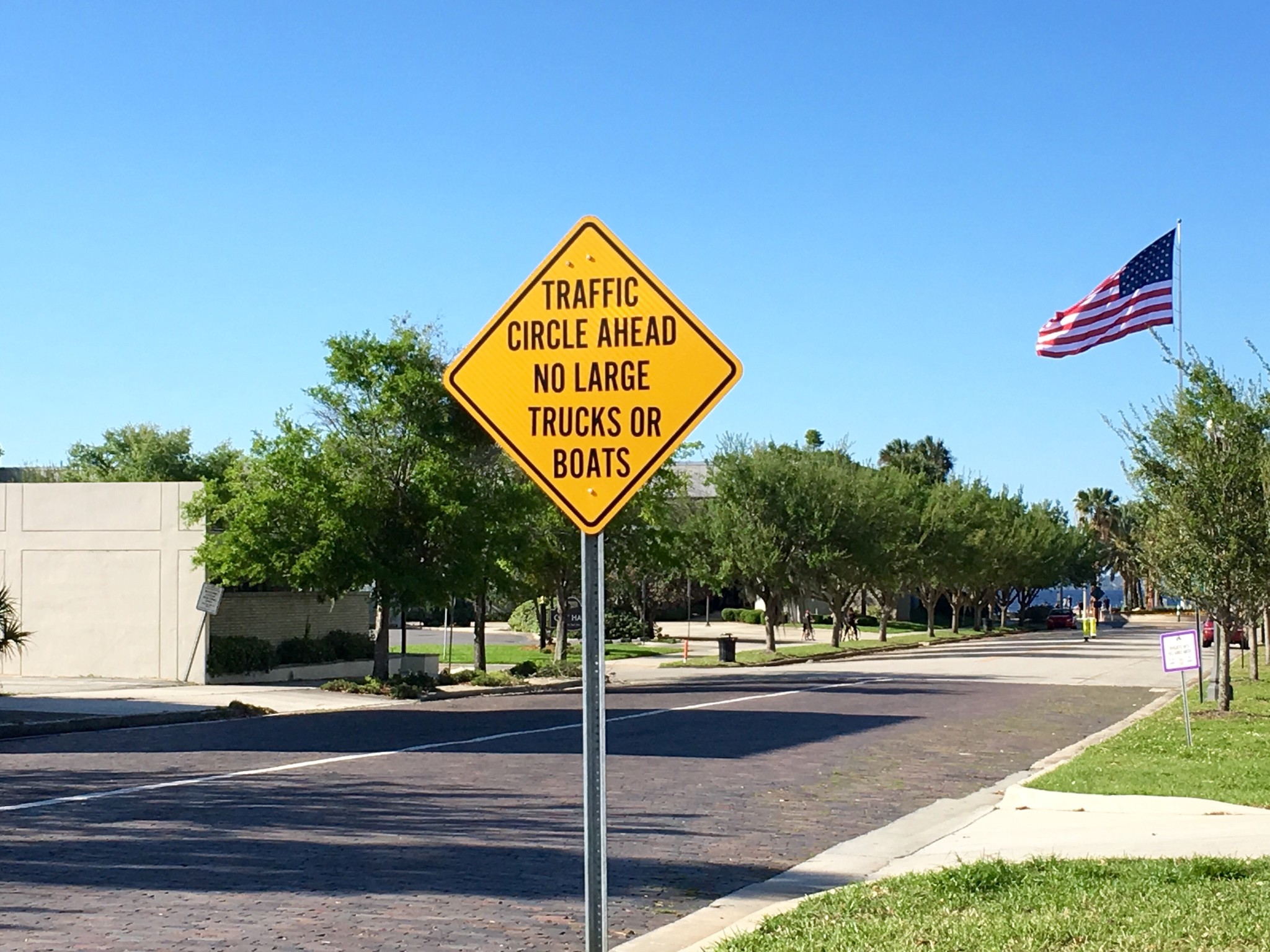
[804, 619, 815, 641]
[847, 625, 861, 642]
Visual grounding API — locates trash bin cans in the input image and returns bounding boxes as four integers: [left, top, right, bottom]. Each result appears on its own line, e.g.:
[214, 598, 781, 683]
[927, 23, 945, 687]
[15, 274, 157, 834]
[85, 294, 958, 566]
[812, 614, 823, 623]
[716, 633, 738, 662]
[982, 618, 993, 631]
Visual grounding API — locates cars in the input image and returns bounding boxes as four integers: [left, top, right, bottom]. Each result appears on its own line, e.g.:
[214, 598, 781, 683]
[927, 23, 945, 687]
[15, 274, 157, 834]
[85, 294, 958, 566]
[1200, 605, 1251, 650]
[1045, 607, 1077, 630]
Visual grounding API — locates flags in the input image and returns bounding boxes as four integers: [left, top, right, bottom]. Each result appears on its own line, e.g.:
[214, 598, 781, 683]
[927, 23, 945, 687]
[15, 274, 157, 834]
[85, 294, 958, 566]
[1035, 227, 1175, 358]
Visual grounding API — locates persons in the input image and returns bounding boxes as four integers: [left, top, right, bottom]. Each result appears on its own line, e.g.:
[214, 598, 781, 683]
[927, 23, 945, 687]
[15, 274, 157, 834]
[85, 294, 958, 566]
[830, 607, 858, 642]
[801, 609, 816, 641]
[1103, 595, 1110, 611]
[1055, 594, 1072, 608]
[1078, 600, 1083, 617]
